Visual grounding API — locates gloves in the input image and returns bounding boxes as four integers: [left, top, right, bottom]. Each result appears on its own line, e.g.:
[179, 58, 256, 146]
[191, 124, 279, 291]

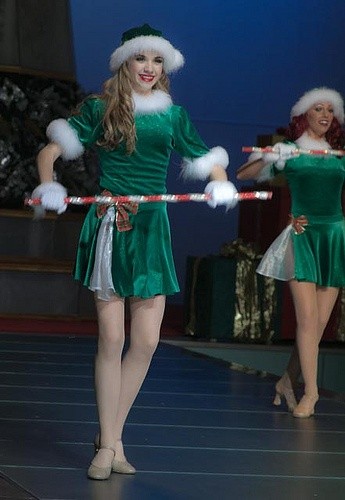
[31, 181, 68, 220]
[203, 180, 239, 214]
[263, 141, 299, 169]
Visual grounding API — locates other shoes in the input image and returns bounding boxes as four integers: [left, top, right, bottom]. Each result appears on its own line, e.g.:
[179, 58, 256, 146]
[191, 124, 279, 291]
[93, 433, 137, 474]
[86, 446, 116, 479]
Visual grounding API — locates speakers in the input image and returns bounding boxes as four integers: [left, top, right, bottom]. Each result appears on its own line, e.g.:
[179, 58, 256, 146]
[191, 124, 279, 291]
[182, 253, 237, 342]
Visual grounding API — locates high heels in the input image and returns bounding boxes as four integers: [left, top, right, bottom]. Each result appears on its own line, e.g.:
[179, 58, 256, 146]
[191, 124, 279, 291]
[292, 385, 319, 418]
[274, 379, 298, 412]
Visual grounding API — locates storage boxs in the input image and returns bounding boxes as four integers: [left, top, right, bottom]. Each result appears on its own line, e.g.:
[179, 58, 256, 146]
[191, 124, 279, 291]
[239, 186, 345, 342]
[187, 256, 283, 343]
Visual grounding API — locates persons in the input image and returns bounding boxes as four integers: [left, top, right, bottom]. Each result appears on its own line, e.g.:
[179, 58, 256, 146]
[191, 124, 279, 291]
[237, 88, 345, 417]
[38, 27, 237, 479]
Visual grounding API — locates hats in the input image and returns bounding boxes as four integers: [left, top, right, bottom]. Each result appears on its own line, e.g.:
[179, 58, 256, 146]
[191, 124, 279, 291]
[109, 22, 185, 74]
[290, 86, 344, 126]
